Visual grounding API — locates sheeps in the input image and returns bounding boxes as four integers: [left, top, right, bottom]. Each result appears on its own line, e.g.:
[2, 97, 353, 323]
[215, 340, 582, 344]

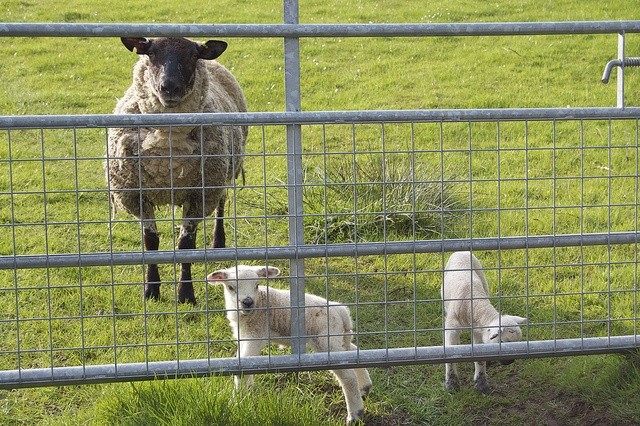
[438, 251, 528, 394]
[206, 264, 373, 425]
[103, 34, 251, 308]
